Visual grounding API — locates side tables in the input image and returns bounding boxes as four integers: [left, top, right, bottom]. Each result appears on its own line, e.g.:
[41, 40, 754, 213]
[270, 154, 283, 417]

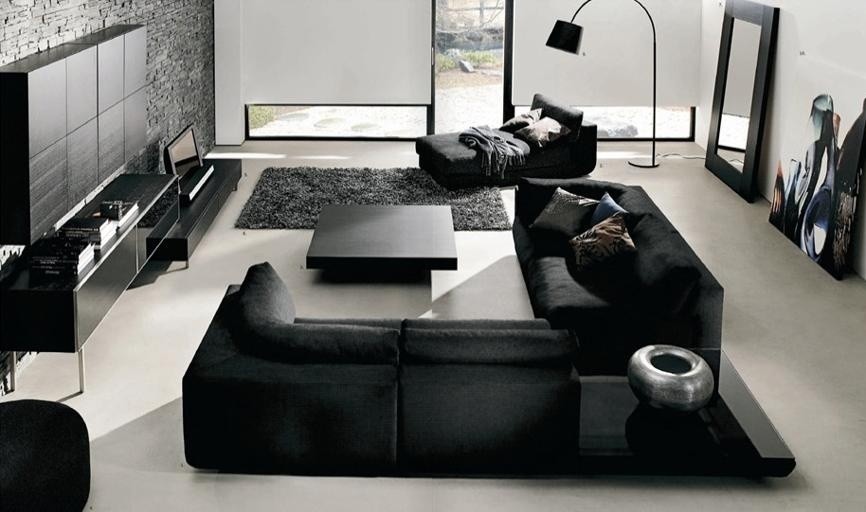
[578, 348, 798, 478]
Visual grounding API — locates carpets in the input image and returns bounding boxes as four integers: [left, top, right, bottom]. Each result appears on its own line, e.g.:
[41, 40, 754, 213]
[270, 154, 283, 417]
[235, 168, 512, 231]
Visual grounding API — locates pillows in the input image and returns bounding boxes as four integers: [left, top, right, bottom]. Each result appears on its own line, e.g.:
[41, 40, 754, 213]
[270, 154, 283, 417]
[592, 191, 628, 225]
[513, 116, 571, 149]
[567, 211, 636, 273]
[529, 186, 598, 238]
[500, 108, 543, 133]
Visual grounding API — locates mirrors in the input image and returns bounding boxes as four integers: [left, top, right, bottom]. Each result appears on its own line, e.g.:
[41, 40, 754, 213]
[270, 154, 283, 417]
[705, 0, 781, 204]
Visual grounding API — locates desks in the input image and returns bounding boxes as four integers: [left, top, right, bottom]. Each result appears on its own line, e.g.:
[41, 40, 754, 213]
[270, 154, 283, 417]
[152, 158, 243, 268]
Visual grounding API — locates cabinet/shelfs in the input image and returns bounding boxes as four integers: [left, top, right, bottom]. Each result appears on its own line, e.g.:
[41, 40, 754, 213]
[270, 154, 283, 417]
[1, 174, 181, 394]
[1, 25, 147, 245]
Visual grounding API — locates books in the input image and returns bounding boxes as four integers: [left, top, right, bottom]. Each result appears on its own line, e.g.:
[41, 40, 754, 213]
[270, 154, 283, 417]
[61, 217, 117, 250]
[27, 237, 95, 276]
[88, 198, 140, 228]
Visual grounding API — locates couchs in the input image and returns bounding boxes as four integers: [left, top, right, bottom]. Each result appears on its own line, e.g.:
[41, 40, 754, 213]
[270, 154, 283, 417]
[513, 177, 724, 349]
[182, 261, 582, 476]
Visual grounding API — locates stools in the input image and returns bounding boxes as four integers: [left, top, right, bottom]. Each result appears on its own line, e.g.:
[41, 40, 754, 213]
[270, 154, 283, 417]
[0, 397, 91, 512]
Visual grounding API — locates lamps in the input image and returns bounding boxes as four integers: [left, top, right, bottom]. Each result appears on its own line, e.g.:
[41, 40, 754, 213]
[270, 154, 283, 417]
[546, 0, 662, 168]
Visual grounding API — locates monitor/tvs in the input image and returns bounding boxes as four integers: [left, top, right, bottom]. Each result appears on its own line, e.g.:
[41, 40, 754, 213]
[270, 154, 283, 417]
[163, 123, 215, 204]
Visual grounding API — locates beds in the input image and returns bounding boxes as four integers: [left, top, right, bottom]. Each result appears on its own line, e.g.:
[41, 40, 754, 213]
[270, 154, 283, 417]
[415, 93, 597, 190]
